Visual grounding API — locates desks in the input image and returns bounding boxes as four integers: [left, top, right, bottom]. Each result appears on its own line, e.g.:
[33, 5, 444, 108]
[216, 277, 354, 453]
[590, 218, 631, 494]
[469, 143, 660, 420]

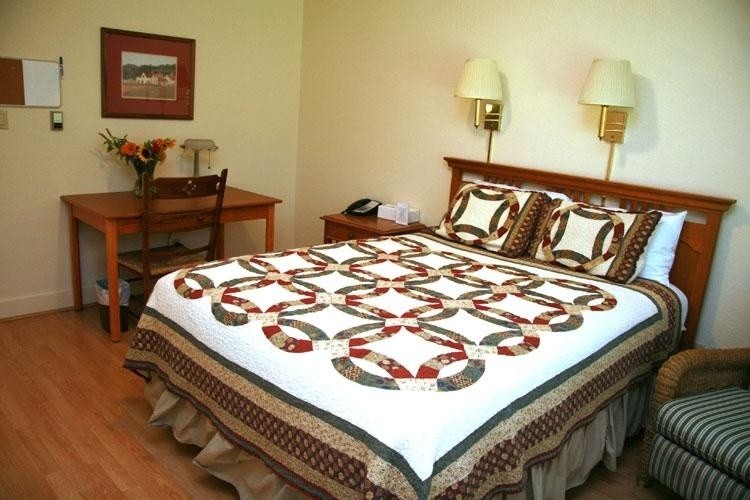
[61, 186, 282, 342]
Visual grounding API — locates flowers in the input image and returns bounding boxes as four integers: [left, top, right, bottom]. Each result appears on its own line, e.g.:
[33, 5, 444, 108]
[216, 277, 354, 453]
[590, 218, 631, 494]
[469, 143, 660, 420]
[98, 128, 176, 173]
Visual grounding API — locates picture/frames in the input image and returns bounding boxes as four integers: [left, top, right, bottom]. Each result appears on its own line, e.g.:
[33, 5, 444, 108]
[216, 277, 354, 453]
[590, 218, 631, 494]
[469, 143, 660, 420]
[100, 27, 196, 121]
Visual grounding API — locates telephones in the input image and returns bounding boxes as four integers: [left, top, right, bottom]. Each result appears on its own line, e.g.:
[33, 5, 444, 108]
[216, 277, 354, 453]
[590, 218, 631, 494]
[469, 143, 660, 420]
[346, 198, 382, 217]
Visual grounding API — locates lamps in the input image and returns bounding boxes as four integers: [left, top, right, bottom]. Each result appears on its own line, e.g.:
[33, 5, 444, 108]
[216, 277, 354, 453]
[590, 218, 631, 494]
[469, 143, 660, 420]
[453, 58, 503, 163]
[180, 139, 219, 178]
[578, 58, 635, 181]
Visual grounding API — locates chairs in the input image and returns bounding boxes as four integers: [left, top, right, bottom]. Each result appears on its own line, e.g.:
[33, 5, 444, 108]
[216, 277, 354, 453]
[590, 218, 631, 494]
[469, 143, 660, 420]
[636, 347, 750, 500]
[118, 169, 228, 320]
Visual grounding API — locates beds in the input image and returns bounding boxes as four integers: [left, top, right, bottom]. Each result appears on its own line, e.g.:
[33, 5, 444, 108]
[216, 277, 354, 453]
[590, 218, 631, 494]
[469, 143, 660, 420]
[123, 157, 737, 500]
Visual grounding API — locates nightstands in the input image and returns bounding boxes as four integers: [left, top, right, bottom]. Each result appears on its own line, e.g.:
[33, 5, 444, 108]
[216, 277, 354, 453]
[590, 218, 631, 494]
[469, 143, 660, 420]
[320, 213, 426, 244]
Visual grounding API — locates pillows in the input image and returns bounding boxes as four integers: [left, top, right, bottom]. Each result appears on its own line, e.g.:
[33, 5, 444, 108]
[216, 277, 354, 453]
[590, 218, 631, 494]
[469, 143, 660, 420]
[423, 178, 688, 288]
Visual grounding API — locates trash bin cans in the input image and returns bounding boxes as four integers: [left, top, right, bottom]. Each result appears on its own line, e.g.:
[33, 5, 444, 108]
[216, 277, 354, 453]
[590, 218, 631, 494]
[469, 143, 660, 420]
[96, 278, 130, 334]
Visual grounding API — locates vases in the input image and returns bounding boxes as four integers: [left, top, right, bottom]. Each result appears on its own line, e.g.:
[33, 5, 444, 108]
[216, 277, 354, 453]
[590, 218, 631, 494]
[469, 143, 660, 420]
[132, 160, 156, 198]
